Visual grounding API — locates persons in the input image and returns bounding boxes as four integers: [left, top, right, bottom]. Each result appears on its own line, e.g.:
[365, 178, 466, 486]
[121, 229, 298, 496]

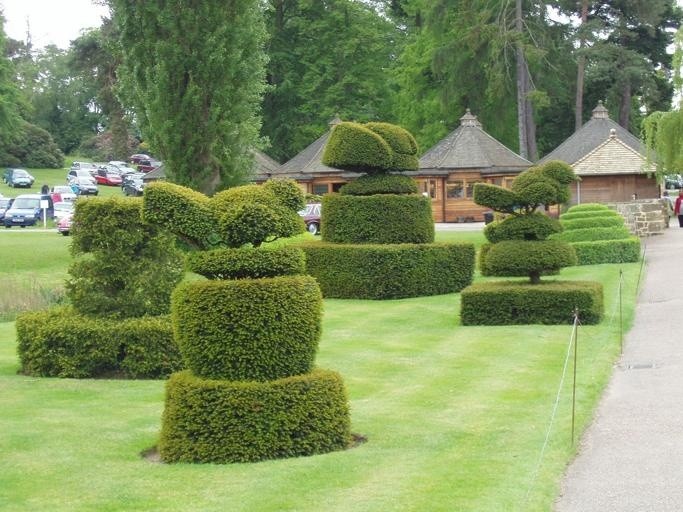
[662, 192, 683, 227]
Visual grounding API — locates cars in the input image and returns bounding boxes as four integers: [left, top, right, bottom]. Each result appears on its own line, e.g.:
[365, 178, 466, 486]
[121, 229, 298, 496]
[0, 185, 78, 236]
[297, 203, 322, 236]
[663, 173, 683, 189]
[66, 153, 160, 197]
[2, 168, 34, 188]
